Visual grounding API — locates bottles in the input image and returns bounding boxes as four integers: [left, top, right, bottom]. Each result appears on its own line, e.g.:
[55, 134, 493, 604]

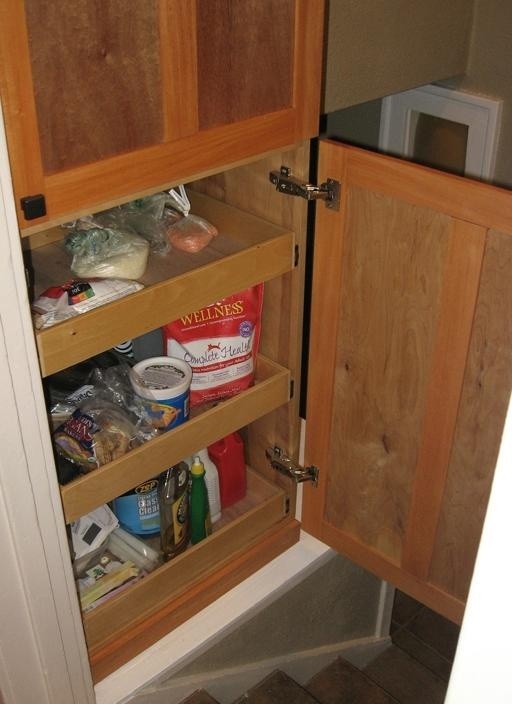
[156, 461, 190, 562]
[187, 455, 211, 545]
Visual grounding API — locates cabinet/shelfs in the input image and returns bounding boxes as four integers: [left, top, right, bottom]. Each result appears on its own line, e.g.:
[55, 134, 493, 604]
[0, 2, 512, 701]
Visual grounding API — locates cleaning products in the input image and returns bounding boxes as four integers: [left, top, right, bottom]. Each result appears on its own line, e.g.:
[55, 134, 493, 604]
[207, 432, 247, 507]
[189, 456, 213, 544]
